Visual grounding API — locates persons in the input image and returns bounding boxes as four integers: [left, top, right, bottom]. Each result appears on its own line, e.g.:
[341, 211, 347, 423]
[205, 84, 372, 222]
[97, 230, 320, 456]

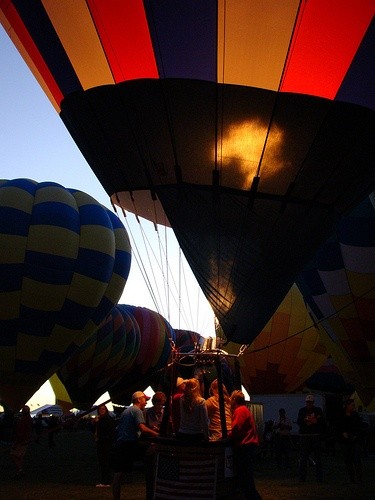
[143, 375, 233, 444]
[0, 402, 116, 489]
[224, 389, 265, 500]
[110, 390, 161, 500]
[262, 393, 367, 486]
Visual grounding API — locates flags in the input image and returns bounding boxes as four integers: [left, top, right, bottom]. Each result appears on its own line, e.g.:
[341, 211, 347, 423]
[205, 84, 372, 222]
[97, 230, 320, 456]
[153, 455, 218, 500]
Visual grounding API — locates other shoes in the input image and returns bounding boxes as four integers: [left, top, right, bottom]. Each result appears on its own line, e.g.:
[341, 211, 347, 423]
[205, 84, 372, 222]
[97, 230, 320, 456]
[13, 470, 24, 480]
[96, 483, 112, 488]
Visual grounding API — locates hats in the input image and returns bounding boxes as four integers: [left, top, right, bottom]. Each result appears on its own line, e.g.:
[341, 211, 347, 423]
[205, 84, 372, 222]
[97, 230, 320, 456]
[304, 395, 315, 402]
[132, 391, 150, 401]
